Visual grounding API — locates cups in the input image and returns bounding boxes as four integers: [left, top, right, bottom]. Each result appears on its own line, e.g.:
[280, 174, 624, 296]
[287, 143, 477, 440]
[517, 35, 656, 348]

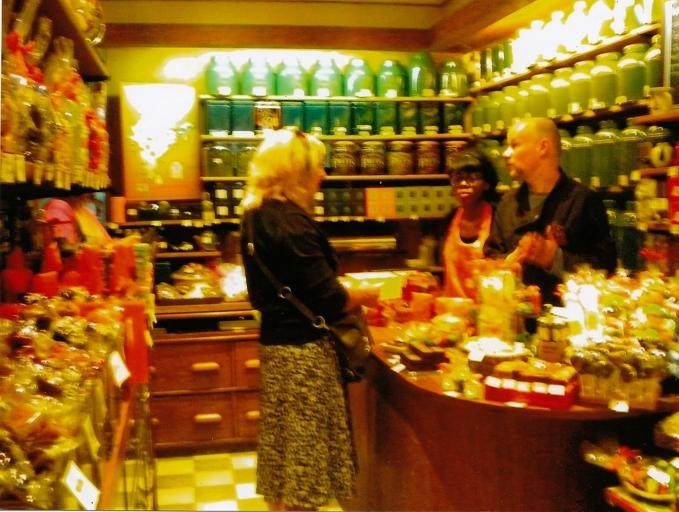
[412, 292, 433, 323]
[406, 236, 439, 269]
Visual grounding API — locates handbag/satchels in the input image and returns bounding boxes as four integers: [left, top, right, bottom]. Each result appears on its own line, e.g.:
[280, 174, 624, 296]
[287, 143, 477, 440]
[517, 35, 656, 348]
[334, 306, 376, 387]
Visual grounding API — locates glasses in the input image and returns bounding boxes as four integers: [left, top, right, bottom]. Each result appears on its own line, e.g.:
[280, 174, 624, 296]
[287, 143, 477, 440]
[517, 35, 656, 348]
[450, 170, 485, 189]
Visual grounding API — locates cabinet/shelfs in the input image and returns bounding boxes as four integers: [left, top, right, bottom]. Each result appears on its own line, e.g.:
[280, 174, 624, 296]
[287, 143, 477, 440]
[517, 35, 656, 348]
[0, 0, 679, 512]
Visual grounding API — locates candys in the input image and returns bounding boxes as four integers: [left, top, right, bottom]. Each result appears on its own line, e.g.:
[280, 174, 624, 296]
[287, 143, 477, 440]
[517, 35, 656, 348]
[569, 340, 666, 400]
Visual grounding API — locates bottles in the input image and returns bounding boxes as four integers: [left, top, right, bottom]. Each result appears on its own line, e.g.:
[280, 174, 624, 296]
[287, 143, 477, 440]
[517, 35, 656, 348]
[472, 0, 678, 274]
[536, 316, 569, 361]
[204, 50, 466, 179]
[125, 192, 215, 220]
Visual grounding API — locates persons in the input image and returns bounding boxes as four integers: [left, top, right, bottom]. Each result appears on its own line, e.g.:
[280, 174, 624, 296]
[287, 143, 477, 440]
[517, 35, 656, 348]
[440, 146, 498, 298]
[240, 128, 370, 512]
[484, 116, 615, 303]
